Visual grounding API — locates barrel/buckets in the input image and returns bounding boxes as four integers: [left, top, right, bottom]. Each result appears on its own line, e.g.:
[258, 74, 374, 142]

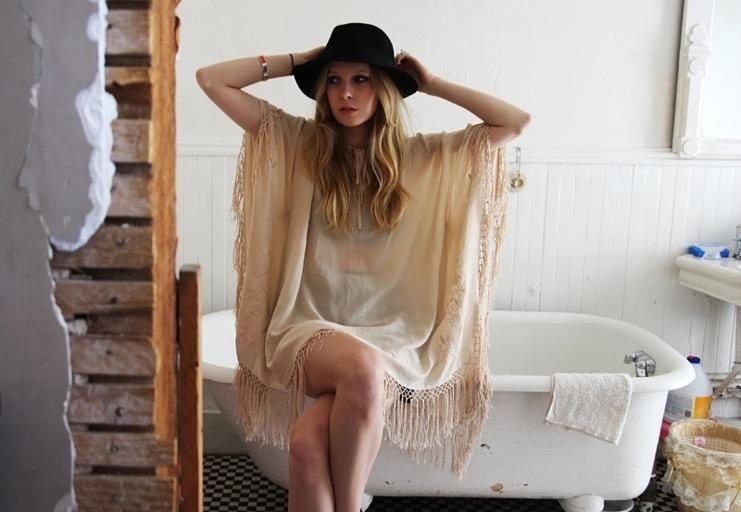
[662, 356, 715, 425]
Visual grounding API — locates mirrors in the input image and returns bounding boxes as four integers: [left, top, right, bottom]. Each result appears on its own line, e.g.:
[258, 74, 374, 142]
[669, 1, 741, 162]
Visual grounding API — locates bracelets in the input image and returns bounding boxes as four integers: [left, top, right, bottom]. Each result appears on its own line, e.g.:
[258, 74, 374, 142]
[287, 51, 295, 69]
[256, 54, 270, 81]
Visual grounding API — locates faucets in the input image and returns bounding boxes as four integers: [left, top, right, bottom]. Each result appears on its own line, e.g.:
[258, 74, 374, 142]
[730, 223, 741, 260]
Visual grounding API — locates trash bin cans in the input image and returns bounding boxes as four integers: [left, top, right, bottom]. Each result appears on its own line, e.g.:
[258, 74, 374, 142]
[668, 419, 741, 512]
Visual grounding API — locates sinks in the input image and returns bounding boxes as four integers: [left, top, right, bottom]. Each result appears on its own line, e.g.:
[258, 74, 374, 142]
[674, 249, 741, 309]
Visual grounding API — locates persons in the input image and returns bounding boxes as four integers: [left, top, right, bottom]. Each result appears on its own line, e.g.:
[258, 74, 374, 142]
[191, 22, 532, 512]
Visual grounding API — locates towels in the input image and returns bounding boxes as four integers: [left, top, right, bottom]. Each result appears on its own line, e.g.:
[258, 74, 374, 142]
[544, 370, 632, 445]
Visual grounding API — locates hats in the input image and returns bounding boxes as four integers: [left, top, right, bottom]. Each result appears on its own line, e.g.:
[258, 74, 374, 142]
[292, 23, 420, 98]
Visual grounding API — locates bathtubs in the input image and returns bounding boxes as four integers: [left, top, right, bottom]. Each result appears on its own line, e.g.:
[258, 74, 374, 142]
[199, 308, 696, 512]
